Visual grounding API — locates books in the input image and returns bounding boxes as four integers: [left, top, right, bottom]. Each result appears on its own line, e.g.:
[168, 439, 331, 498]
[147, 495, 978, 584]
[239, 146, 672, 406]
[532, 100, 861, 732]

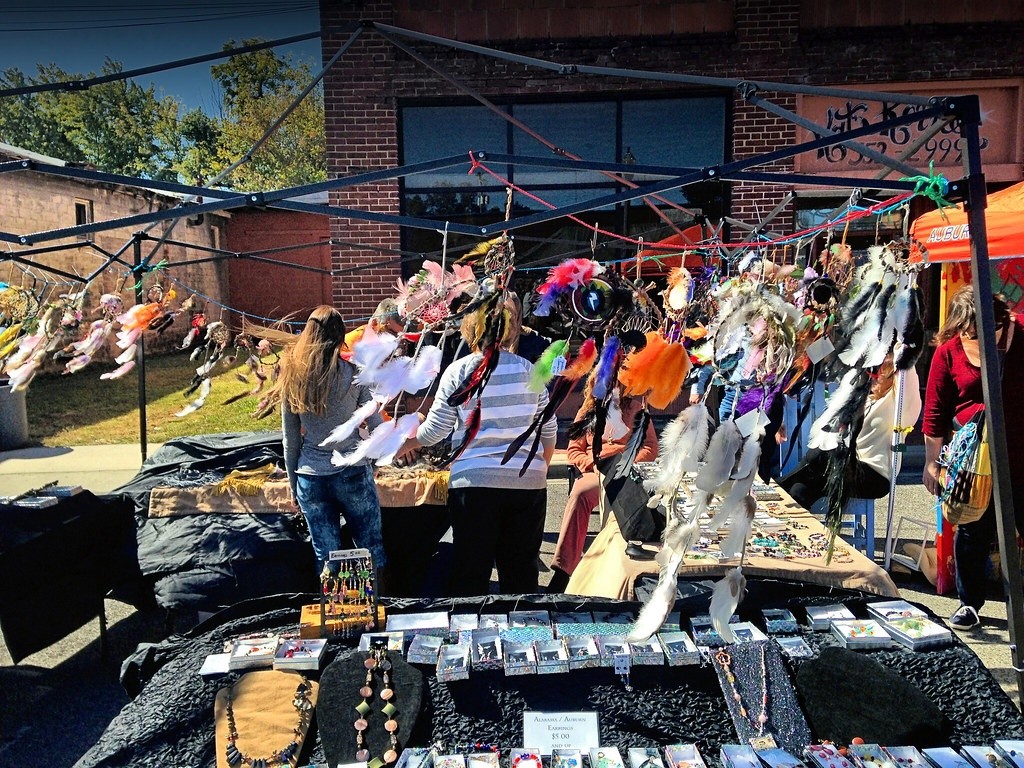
[16, 486, 82, 509]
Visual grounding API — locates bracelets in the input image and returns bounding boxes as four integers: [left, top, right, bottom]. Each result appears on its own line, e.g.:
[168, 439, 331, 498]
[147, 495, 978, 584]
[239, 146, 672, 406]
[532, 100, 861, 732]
[747, 502, 854, 563]
[245, 646, 275, 655]
[513, 753, 541, 768]
[455, 743, 501, 762]
[860, 754, 883, 768]
[808, 744, 848, 768]
[848, 626, 874, 637]
[284, 647, 312, 658]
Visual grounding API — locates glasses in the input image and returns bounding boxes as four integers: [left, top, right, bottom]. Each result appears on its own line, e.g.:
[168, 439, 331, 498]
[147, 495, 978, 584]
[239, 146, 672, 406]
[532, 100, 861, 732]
[966, 323, 1004, 340]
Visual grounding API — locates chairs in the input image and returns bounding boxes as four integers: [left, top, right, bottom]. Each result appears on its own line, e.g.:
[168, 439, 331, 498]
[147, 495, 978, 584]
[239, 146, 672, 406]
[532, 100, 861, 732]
[779, 382, 874, 562]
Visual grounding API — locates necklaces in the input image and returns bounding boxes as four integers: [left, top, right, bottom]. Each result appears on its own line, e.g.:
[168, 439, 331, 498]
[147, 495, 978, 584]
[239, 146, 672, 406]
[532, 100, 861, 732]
[717, 643, 777, 751]
[354, 649, 397, 768]
[322, 558, 375, 639]
[226, 675, 312, 768]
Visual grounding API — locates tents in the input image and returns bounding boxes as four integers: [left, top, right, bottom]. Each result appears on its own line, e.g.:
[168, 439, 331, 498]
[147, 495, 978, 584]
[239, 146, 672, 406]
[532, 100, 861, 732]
[885, 181, 1024, 568]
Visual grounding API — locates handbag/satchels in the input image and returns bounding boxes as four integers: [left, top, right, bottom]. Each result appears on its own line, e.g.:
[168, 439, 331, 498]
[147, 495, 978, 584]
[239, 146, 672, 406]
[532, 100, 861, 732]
[937, 421, 993, 525]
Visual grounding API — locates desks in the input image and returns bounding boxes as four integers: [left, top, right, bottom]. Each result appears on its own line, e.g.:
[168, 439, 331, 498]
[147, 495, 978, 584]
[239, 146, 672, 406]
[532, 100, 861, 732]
[565, 461, 901, 601]
[0, 494, 144, 666]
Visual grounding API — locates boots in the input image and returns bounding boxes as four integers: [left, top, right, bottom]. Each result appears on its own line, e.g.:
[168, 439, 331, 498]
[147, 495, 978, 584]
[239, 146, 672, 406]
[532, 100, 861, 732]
[775, 453, 815, 491]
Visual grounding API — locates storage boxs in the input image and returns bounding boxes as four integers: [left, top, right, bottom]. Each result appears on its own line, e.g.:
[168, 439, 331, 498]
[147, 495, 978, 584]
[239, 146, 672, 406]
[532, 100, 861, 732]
[394, 740, 1024, 768]
[198, 601, 953, 683]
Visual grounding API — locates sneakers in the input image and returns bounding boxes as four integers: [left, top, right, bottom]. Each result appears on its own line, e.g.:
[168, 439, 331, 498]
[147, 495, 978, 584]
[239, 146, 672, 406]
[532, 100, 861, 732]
[948, 606, 981, 630]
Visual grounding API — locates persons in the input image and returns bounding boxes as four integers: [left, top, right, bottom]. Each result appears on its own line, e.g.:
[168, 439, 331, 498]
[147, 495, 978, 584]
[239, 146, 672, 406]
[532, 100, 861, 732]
[546, 365, 658, 594]
[778, 352, 922, 511]
[394, 300, 558, 598]
[692, 350, 785, 444]
[280, 306, 385, 593]
[340, 298, 473, 466]
[921, 285, 1024, 630]
